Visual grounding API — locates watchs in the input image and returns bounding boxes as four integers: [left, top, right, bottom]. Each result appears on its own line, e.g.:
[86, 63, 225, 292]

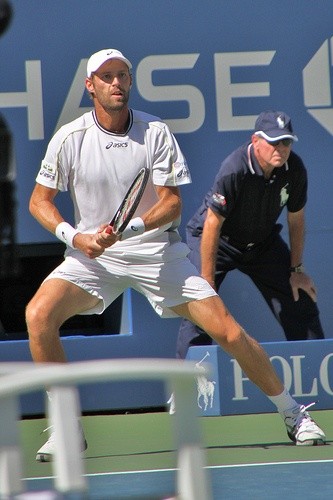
[290, 263, 305, 273]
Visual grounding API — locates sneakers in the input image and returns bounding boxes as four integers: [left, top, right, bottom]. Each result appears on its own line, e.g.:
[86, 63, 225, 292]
[280, 401, 327, 447]
[36, 422, 89, 462]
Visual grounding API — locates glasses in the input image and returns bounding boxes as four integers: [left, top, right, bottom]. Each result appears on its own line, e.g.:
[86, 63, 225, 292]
[268, 139, 292, 146]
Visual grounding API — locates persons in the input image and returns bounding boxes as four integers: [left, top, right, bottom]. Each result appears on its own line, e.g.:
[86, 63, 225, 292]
[167, 110, 327, 416]
[20, 48, 327, 461]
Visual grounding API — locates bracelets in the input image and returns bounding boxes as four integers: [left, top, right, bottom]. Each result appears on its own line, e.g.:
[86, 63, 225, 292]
[119, 218, 145, 241]
[54, 221, 81, 250]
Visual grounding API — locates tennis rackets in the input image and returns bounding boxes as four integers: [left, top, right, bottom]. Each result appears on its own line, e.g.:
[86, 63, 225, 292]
[88, 167, 150, 254]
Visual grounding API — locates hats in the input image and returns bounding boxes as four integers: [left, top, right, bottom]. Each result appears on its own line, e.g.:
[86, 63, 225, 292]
[253, 111, 299, 143]
[85, 49, 132, 77]
